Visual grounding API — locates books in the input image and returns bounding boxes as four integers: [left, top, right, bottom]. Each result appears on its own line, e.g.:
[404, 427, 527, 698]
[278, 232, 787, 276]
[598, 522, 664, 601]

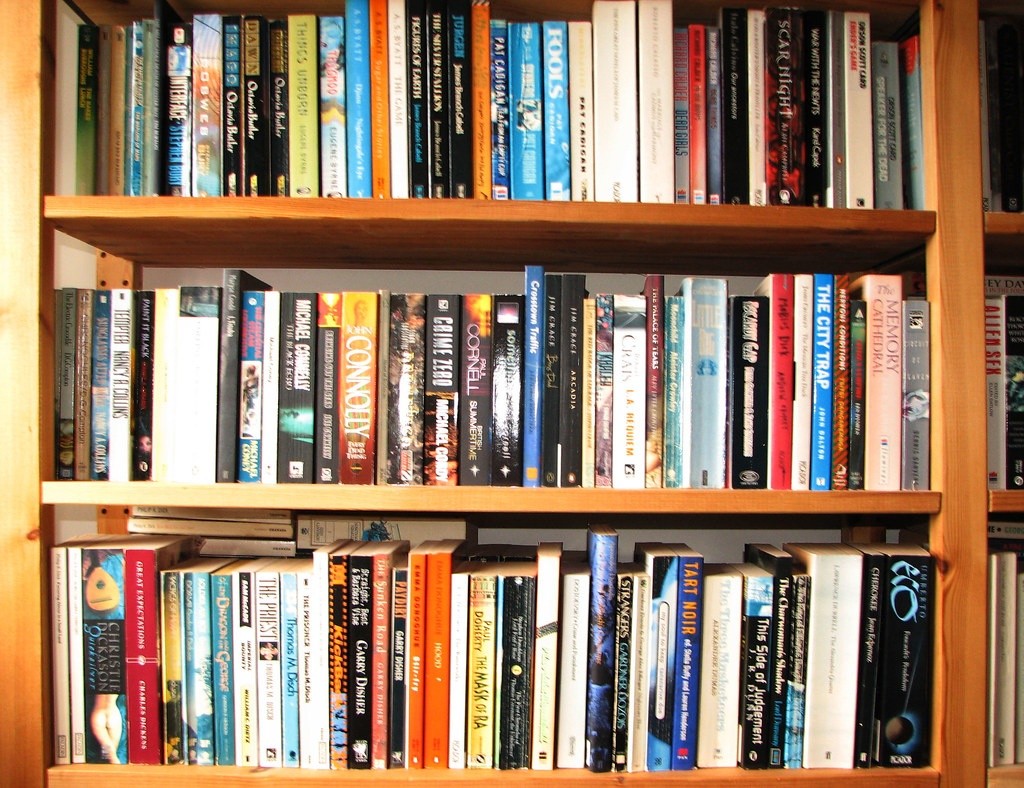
[77, 0, 925, 211]
[51, 504, 936, 769]
[987, 522, 1024, 769]
[54, 265, 929, 491]
[985, 276, 1024, 491]
[980, 18, 1024, 212]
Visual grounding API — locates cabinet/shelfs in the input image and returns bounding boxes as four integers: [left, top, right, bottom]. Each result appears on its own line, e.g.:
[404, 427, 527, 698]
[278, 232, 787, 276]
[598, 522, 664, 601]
[0, 0, 1024, 788]
